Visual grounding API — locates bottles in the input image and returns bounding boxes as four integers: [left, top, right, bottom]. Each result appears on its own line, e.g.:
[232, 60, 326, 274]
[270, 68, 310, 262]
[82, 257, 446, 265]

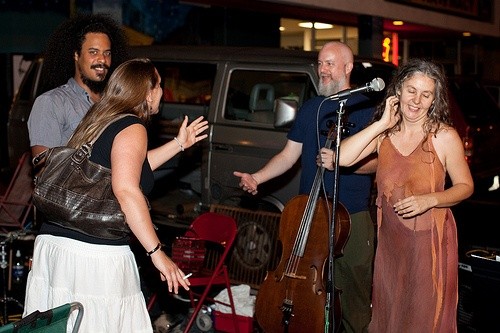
[12, 250, 27, 288]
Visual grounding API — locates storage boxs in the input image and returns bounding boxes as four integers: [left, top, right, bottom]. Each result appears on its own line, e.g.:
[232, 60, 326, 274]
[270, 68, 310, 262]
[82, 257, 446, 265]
[213, 310, 253, 333]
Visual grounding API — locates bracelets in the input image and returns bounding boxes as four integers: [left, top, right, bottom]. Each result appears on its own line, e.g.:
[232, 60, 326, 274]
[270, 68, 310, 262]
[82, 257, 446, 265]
[174, 137, 185, 152]
[146, 243, 159, 256]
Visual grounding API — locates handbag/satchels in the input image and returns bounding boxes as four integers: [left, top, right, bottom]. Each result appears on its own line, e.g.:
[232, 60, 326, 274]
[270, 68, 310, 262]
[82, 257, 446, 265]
[33, 113, 151, 240]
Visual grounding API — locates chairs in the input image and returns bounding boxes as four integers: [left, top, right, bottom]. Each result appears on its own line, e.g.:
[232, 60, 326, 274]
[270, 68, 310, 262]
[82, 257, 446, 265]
[146, 212, 237, 333]
[0, 302, 84, 333]
[245, 83, 275, 124]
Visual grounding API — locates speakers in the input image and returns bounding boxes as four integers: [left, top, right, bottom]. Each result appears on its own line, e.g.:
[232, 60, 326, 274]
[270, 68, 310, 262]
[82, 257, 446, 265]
[456, 257, 500, 333]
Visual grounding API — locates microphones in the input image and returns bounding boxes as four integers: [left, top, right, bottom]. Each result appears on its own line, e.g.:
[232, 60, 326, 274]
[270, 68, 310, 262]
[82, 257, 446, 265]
[329, 78, 385, 102]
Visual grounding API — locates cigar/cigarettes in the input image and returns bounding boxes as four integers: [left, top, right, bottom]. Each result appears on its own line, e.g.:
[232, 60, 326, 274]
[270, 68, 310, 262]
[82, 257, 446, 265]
[182, 273, 192, 280]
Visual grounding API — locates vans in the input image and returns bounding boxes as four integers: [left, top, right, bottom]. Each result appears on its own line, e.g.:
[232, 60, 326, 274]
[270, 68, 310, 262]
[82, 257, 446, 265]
[4, 46, 400, 288]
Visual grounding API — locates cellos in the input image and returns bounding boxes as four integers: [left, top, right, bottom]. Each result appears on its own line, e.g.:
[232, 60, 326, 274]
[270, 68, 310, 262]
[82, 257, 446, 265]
[252, 113, 357, 333]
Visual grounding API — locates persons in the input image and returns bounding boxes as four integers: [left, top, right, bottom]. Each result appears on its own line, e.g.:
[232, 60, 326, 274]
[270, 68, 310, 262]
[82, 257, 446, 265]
[26, 21, 120, 158]
[21, 57, 209, 333]
[332, 59, 475, 333]
[232, 44, 376, 333]
